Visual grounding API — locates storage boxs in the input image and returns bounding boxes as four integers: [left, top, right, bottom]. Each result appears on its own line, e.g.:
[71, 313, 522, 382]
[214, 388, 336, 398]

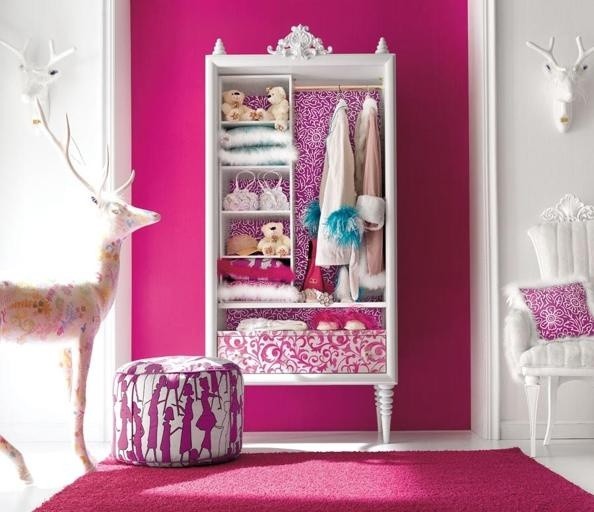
[216, 329, 387, 373]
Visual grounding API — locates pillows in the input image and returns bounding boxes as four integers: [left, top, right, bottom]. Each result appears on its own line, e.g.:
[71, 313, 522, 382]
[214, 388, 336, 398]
[217, 258, 304, 303]
[504, 273, 594, 344]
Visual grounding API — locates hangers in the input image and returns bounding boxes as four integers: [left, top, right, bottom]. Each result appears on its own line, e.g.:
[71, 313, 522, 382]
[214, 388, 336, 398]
[339, 85, 341, 98]
[365, 85, 371, 101]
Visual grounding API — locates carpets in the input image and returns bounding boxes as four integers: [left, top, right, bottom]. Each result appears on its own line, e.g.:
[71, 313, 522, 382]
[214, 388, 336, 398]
[33, 448, 594, 512]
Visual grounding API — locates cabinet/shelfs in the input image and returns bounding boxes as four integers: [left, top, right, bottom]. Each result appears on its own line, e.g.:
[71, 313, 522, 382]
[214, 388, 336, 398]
[205, 23, 399, 443]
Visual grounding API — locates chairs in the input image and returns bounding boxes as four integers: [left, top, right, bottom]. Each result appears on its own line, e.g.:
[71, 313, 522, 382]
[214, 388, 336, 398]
[503, 194, 594, 458]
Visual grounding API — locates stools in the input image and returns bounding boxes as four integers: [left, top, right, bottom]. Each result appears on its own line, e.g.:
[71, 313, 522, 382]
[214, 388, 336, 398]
[114, 356, 245, 468]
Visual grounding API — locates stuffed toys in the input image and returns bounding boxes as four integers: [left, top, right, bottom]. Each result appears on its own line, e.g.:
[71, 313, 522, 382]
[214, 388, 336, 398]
[254, 86, 290, 133]
[256, 222, 290, 256]
[220, 89, 259, 122]
